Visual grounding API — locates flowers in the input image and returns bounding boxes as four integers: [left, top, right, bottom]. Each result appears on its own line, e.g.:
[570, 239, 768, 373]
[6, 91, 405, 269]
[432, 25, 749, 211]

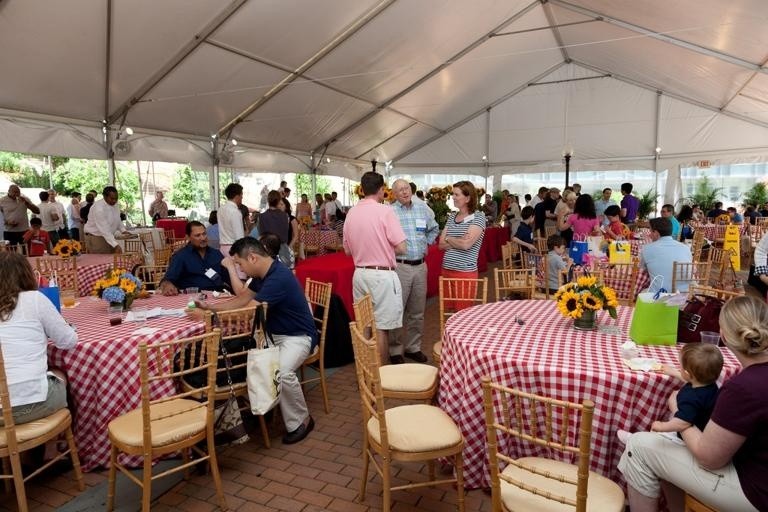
[425, 185, 453, 225]
[554, 276, 618, 320]
[299, 215, 311, 230]
[94, 269, 145, 303]
[477, 188, 487, 206]
[53, 239, 82, 258]
[354, 183, 366, 201]
[381, 183, 395, 204]
[716, 214, 730, 223]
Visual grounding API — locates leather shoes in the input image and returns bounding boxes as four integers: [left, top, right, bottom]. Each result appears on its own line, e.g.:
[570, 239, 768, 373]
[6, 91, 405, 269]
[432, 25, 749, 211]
[42, 454, 85, 475]
[404, 350, 428, 363]
[388, 354, 405, 365]
[280, 414, 315, 445]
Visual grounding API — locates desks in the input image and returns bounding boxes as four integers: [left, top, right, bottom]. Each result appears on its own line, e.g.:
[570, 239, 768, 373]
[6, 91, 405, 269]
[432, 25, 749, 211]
[536, 252, 650, 300]
[115, 227, 165, 272]
[691, 222, 744, 251]
[293, 227, 511, 320]
[628, 227, 652, 253]
[157, 218, 188, 241]
[439, 299, 744, 489]
[45, 291, 259, 474]
[25, 254, 144, 298]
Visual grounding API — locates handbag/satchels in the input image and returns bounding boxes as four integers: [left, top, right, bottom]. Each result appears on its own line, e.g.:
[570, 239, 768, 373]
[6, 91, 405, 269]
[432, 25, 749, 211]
[569, 232, 589, 265]
[247, 304, 283, 416]
[587, 230, 607, 259]
[679, 293, 727, 347]
[629, 274, 678, 347]
[609, 236, 631, 266]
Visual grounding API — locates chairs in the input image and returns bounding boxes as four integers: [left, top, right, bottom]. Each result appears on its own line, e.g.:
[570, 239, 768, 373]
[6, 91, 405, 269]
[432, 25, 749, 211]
[37, 256, 79, 297]
[296, 278, 332, 414]
[350, 321, 466, 512]
[494, 267, 536, 301]
[0, 242, 28, 260]
[0, 346, 85, 512]
[523, 251, 550, 299]
[482, 376, 625, 512]
[434, 276, 488, 367]
[111, 229, 188, 290]
[684, 494, 721, 512]
[175, 301, 272, 450]
[600, 263, 639, 305]
[535, 226, 557, 255]
[350, 294, 438, 418]
[708, 245, 729, 287]
[754, 217, 768, 243]
[671, 261, 712, 293]
[107, 328, 228, 512]
[501, 240, 523, 270]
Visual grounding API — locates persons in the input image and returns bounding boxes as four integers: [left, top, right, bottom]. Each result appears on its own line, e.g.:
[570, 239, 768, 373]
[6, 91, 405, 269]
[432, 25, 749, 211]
[1, 251, 79, 483]
[527, 189, 556, 237]
[343, 171, 404, 366]
[257, 192, 290, 270]
[601, 206, 621, 239]
[31, 191, 57, 250]
[615, 297, 766, 511]
[215, 184, 245, 286]
[676, 204, 696, 235]
[637, 205, 679, 238]
[618, 182, 640, 224]
[539, 237, 572, 297]
[498, 190, 511, 219]
[724, 207, 743, 224]
[314, 193, 325, 225]
[221, 234, 280, 299]
[692, 204, 703, 226]
[157, 220, 235, 297]
[557, 194, 598, 240]
[72, 199, 80, 220]
[66, 192, 81, 254]
[483, 193, 498, 221]
[556, 189, 579, 243]
[332, 192, 342, 221]
[25, 217, 53, 254]
[503, 194, 518, 222]
[82, 189, 95, 208]
[48, 189, 66, 242]
[761, 203, 767, 216]
[206, 210, 223, 249]
[148, 191, 166, 222]
[616, 342, 723, 448]
[747, 227, 767, 295]
[80, 194, 94, 225]
[636, 217, 696, 295]
[237, 200, 250, 235]
[511, 206, 536, 253]
[747, 206, 761, 224]
[524, 195, 535, 209]
[277, 181, 286, 198]
[525, 187, 548, 212]
[279, 187, 296, 222]
[438, 179, 485, 322]
[573, 183, 582, 196]
[0, 184, 40, 249]
[382, 179, 439, 363]
[321, 193, 330, 227]
[276, 199, 298, 271]
[83, 187, 131, 254]
[325, 194, 336, 224]
[297, 194, 311, 224]
[594, 188, 616, 220]
[554, 191, 570, 223]
[707, 201, 727, 225]
[258, 185, 269, 210]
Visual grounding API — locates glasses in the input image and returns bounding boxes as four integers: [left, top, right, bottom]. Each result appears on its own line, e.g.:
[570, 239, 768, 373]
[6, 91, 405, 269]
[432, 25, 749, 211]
[661, 210, 671, 214]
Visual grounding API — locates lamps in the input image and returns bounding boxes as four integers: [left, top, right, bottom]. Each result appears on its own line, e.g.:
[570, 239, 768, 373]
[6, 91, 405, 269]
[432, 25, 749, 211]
[482, 155, 488, 165]
[324, 157, 331, 162]
[120, 128, 133, 140]
[228, 139, 239, 148]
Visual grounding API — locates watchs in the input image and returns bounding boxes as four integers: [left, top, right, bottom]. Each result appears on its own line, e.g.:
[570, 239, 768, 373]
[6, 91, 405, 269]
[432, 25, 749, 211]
[445, 237, 448, 243]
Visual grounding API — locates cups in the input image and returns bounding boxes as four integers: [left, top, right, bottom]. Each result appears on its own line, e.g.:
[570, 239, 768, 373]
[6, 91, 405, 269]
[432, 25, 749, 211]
[61, 290, 76, 309]
[185, 287, 199, 307]
[107, 306, 123, 327]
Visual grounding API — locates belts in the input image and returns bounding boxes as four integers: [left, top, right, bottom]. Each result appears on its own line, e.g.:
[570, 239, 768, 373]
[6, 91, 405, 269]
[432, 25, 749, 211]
[396, 257, 426, 266]
[355, 264, 397, 272]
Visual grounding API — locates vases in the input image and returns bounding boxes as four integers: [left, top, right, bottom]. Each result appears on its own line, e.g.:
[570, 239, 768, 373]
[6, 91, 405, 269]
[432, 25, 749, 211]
[109, 293, 135, 312]
[720, 220, 726, 224]
[572, 307, 599, 330]
[64, 255, 72, 262]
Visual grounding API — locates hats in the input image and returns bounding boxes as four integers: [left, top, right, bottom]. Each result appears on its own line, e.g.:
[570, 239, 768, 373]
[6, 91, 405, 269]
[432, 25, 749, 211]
[548, 188, 560, 193]
